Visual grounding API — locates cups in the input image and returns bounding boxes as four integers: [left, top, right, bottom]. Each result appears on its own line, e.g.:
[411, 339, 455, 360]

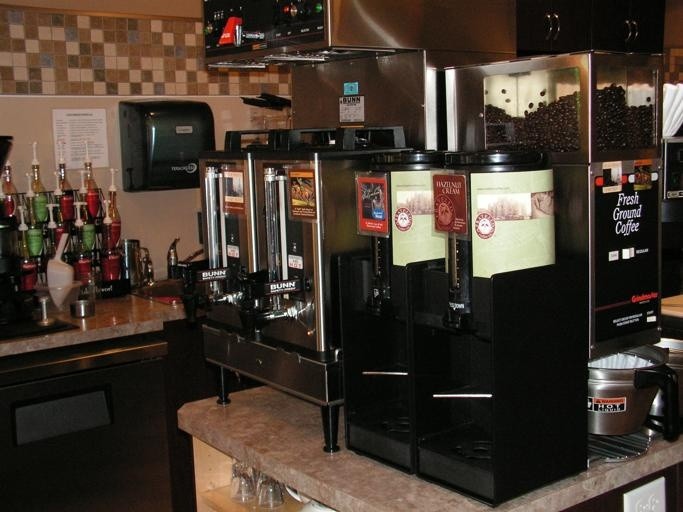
[586, 341, 683, 443]
[120, 239, 150, 290]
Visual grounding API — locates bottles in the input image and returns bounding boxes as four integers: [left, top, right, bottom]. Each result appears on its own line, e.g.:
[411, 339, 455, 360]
[0, 140, 121, 292]
